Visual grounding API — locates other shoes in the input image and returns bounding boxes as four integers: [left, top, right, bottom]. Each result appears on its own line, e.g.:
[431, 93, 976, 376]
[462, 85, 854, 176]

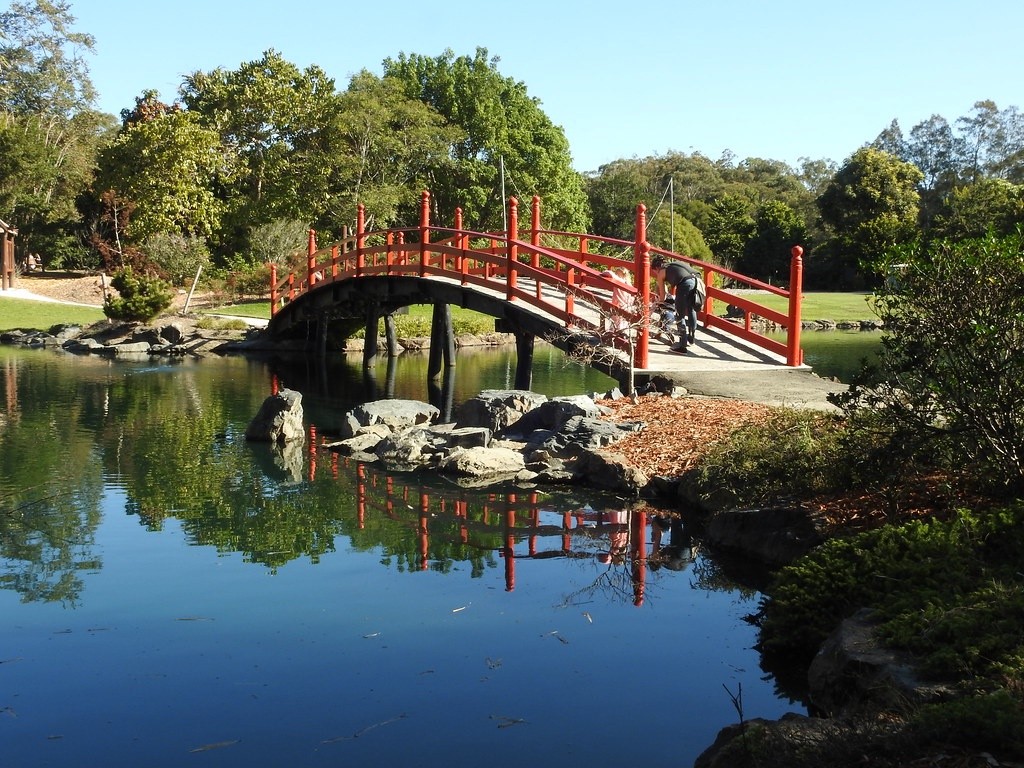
[658, 322, 662, 327]
[686, 333, 694, 344]
[663, 326, 668, 331]
[670, 346, 687, 355]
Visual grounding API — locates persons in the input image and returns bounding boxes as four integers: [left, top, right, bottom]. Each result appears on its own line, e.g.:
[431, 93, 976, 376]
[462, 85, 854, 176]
[651, 254, 702, 355]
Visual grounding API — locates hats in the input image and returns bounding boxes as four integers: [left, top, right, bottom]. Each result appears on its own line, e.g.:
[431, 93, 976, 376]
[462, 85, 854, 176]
[315, 270, 322, 281]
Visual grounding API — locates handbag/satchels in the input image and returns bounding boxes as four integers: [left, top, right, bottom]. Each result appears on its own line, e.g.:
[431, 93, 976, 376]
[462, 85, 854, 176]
[689, 288, 705, 312]
